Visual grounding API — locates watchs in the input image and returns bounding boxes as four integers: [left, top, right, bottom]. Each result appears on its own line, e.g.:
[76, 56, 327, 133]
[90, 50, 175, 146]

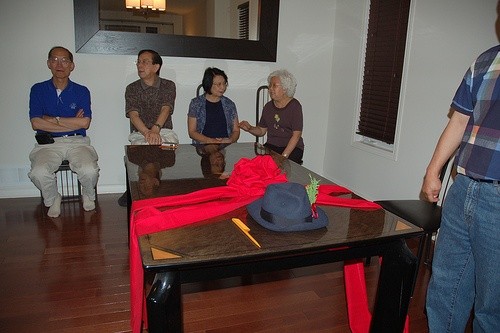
[283, 152, 289, 158]
[154, 123, 161, 130]
[55, 116, 61, 126]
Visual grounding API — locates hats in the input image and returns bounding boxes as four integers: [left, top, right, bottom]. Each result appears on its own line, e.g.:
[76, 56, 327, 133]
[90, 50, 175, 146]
[247, 182, 328, 232]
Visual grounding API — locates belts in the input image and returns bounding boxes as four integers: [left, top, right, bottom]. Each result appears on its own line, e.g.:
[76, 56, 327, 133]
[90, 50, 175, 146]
[53, 133, 81, 137]
[466, 176, 499, 184]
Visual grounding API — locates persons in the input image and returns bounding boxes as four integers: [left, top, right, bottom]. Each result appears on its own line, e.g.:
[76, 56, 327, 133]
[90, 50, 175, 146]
[419, 0, 500, 333]
[187, 67, 240, 143]
[125, 49, 179, 146]
[125, 145, 176, 195]
[238, 69, 304, 165]
[28, 46, 99, 218]
[191, 143, 233, 179]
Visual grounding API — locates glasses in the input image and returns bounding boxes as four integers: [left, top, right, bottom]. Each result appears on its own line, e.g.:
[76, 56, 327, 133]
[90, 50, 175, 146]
[213, 82, 228, 87]
[135, 60, 155, 65]
[51, 57, 72, 64]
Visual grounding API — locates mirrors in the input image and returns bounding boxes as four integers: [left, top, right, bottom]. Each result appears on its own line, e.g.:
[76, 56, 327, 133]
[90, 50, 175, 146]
[73, 0, 281, 62]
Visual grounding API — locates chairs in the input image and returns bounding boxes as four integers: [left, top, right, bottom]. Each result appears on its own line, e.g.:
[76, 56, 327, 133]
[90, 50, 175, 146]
[41, 160, 97, 212]
[257, 84, 270, 143]
[364, 157, 455, 297]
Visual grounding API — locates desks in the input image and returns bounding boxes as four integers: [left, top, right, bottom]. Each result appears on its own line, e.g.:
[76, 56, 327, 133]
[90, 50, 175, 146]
[124, 142, 425, 333]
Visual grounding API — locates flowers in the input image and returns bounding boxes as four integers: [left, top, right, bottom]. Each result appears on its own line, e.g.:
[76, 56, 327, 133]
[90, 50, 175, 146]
[305, 172, 322, 219]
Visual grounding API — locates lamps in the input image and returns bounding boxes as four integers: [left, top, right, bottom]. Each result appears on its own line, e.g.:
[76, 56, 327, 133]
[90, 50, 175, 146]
[125, 0, 167, 19]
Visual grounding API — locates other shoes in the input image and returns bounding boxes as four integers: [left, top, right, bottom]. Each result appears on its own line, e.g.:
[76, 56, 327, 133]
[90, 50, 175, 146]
[119, 192, 128, 207]
